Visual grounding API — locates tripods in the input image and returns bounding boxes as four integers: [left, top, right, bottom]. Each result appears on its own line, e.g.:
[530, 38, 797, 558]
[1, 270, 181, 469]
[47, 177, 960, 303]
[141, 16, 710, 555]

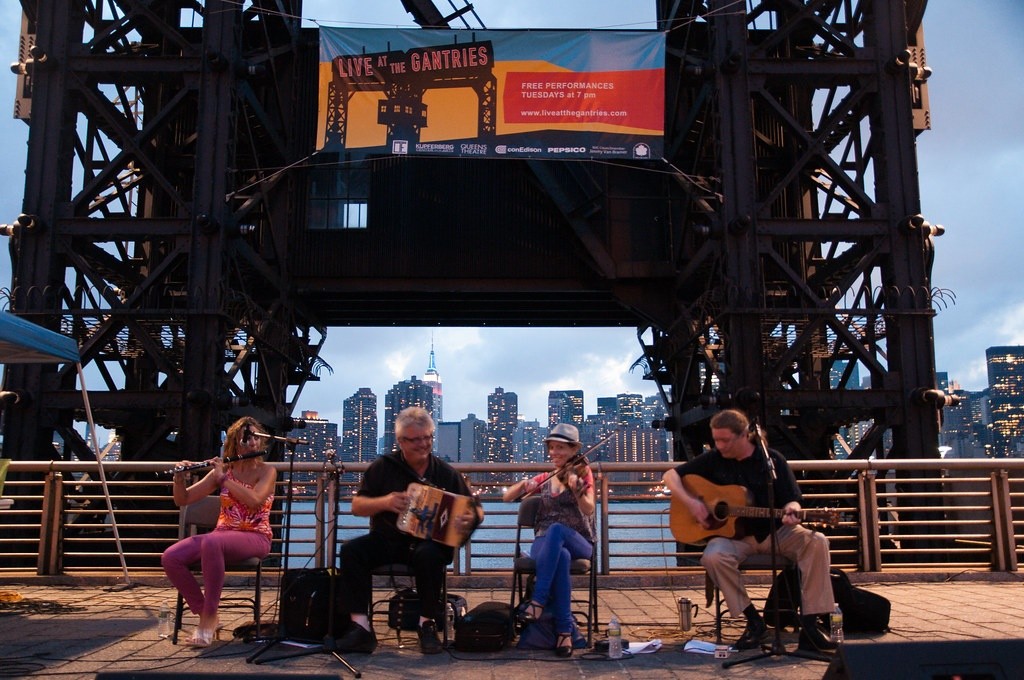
[720, 422, 831, 670]
[245, 429, 362, 678]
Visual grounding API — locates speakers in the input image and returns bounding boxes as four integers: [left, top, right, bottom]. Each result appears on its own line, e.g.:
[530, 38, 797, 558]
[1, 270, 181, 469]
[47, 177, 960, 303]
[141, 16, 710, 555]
[821, 637, 1024, 680]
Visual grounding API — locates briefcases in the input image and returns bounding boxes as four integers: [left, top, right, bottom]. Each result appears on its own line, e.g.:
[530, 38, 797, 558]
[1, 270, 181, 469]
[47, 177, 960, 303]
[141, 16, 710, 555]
[455, 601, 520, 652]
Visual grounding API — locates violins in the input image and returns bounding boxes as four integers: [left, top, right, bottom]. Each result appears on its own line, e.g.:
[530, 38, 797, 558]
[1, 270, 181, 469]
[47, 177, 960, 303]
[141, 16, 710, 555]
[556, 451, 593, 498]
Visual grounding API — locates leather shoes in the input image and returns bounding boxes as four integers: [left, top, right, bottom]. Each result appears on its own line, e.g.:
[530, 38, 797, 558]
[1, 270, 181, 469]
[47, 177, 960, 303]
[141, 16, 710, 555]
[731, 627, 771, 650]
[798, 629, 839, 651]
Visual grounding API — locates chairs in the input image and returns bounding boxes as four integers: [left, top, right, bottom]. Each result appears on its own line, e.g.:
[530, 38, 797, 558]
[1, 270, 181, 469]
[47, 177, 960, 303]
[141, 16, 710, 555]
[369, 546, 455, 646]
[716, 554, 802, 645]
[510, 496, 599, 649]
[173, 496, 262, 645]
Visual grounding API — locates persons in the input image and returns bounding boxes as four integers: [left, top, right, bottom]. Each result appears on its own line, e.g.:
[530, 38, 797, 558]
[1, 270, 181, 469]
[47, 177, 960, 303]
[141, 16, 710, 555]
[503, 423, 599, 657]
[663, 410, 841, 652]
[161, 416, 277, 647]
[336, 407, 485, 654]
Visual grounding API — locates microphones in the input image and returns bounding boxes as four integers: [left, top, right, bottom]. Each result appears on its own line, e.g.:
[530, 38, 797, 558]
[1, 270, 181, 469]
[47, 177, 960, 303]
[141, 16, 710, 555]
[746, 417, 759, 433]
[240, 425, 252, 447]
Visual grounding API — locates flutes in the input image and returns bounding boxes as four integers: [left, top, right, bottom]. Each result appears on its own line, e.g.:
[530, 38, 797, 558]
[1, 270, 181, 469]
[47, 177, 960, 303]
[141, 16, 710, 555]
[154, 449, 268, 478]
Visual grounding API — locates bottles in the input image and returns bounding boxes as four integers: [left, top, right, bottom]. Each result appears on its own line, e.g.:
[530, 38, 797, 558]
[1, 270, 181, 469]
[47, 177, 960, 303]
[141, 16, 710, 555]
[830, 604, 844, 644]
[445, 603, 454, 640]
[608, 617, 622, 658]
[105, 514, 112, 531]
[158, 597, 170, 636]
[678, 597, 698, 631]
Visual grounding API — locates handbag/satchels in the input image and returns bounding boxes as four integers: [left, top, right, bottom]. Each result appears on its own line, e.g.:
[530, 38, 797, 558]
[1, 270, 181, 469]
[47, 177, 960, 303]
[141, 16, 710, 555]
[763, 564, 892, 636]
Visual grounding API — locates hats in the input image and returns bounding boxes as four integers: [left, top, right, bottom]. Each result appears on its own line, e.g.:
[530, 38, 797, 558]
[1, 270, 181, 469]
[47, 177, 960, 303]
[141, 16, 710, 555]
[543, 423, 582, 448]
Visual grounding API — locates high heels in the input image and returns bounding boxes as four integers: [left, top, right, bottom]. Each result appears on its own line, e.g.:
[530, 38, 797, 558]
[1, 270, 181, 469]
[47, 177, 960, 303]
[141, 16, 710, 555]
[192, 617, 223, 645]
[522, 604, 543, 622]
[556, 633, 573, 658]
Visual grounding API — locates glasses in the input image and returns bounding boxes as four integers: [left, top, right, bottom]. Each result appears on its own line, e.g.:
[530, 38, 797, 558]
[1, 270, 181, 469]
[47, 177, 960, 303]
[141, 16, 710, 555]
[404, 434, 434, 443]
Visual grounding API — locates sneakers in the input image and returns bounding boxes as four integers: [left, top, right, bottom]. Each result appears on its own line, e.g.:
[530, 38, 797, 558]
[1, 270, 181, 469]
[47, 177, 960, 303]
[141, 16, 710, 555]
[417, 619, 443, 654]
[323, 621, 378, 653]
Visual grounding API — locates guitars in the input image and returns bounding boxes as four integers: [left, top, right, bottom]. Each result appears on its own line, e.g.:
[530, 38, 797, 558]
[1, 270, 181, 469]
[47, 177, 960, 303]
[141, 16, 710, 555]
[667, 473, 843, 547]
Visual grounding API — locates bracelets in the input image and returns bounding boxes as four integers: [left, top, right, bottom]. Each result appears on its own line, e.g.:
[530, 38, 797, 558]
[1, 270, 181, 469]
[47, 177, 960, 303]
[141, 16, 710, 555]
[218, 475, 229, 487]
